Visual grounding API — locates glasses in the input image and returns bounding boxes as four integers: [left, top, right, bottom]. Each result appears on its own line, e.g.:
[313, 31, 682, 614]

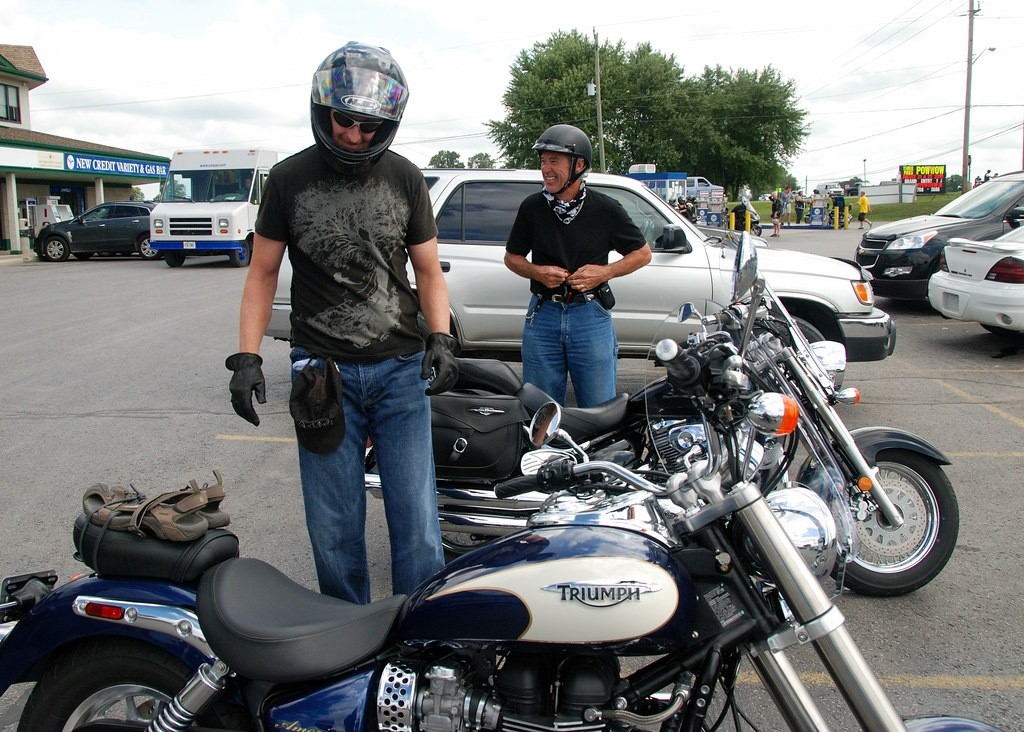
[330, 108, 384, 133]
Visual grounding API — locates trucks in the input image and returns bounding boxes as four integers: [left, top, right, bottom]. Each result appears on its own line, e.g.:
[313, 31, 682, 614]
[817, 183, 844, 197]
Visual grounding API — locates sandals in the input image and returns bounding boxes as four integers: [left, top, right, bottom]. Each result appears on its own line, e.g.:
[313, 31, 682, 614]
[83, 479, 209, 541]
[110, 469, 230, 528]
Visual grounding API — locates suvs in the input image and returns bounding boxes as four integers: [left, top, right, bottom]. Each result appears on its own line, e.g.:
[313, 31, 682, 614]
[852, 171, 1024, 299]
[33, 200, 165, 261]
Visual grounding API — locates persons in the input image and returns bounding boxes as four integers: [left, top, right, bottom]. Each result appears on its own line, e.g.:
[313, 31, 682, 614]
[224, 41, 460, 606]
[810, 190, 825, 206]
[793, 191, 810, 225]
[769, 191, 781, 237]
[984, 169, 992, 182]
[850, 191, 873, 229]
[502, 123, 655, 409]
[779, 187, 794, 228]
[239, 177, 257, 202]
[667, 196, 698, 224]
[721, 201, 747, 230]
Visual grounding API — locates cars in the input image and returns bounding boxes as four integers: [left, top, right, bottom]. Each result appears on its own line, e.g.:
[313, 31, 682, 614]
[262, 170, 898, 410]
[652, 177, 725, 203]
[758, 194, 772, 201]
[790, 190, 806, 199]
[927, 223, 1024, 341]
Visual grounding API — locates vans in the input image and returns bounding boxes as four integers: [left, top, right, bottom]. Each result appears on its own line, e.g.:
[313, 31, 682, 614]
[148, 149, 288, 268]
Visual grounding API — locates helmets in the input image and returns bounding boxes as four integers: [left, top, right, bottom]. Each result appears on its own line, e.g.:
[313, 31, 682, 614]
[687, 195, 696, 203]
[531, 124, 592, 171]
[311, 41, 409, 175]
[669, 197, 676, 205]
[678, 196, 685, 205]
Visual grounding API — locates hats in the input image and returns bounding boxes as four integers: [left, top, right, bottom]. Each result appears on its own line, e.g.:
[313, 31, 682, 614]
[289, 355, 345, 454]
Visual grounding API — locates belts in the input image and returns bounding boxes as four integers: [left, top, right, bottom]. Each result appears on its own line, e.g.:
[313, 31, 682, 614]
[533, 292, 599, 304]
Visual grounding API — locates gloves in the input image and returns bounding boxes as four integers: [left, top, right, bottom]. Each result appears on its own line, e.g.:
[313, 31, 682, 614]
[420, 332, 462, 396]
[226, 352, 266, 427]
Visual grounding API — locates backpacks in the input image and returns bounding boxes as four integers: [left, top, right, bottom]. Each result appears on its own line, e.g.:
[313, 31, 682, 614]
[780, 201, 786, 215]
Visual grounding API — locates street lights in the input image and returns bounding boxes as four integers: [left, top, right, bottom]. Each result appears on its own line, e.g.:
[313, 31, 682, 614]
[863, 159, 867, 186]
[961, 47, 995, 196]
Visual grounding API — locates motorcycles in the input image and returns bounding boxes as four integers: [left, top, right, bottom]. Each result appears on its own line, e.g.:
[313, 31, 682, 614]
[669, 194, 698, 225]
[352, 230, 959, 598]
[1, 331, 1005, 732]
[726, 196, 762, 236]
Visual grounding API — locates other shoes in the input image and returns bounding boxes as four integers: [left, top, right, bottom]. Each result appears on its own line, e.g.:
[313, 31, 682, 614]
[770, 233, 780, 238]
[858, 226, 864, 229]
[870, 222, 873, 228]
